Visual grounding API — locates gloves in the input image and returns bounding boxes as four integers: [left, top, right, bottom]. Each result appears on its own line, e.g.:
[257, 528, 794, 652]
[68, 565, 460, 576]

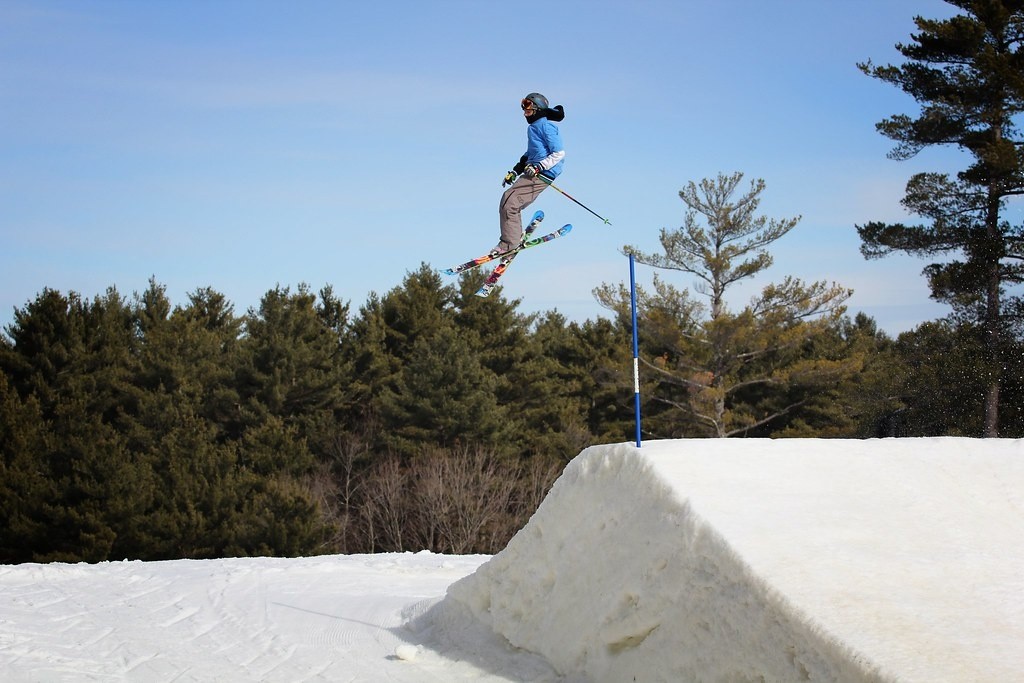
[502, 171, 516, 188]
[524, 163, 544, 179]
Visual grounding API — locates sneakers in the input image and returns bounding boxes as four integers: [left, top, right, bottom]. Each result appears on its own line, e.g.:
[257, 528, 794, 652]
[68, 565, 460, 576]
[500, 254, 512, 264]
[490, 246, 507, 259]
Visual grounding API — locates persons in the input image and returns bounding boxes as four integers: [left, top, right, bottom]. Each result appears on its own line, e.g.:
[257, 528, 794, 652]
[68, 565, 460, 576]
[491, 93, 565, 264]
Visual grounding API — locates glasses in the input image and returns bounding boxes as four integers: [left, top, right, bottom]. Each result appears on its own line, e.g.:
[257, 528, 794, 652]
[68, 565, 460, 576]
[521, 102, 533, 110]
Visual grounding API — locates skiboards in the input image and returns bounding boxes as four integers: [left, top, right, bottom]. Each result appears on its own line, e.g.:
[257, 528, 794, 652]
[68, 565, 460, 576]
[438, 208, 573, 298]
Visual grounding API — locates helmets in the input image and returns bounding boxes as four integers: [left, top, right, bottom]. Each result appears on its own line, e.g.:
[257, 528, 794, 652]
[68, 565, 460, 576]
[525, 93, 549, 110]
